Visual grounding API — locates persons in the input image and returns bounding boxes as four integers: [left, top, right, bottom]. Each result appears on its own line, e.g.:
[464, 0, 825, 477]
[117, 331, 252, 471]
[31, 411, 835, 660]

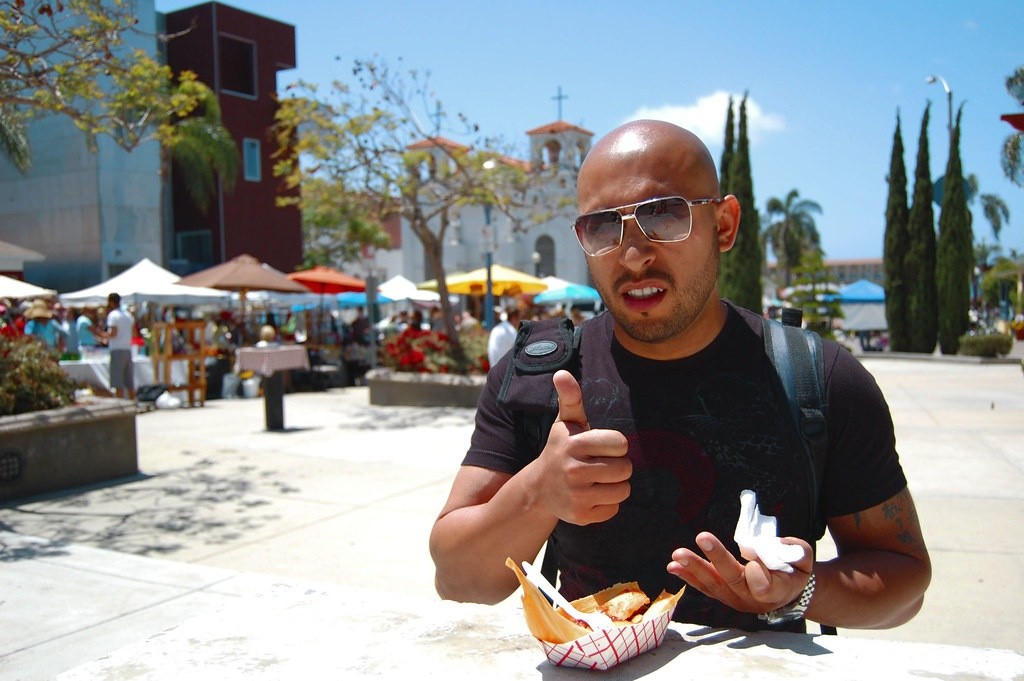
[430, 118, 932, 636]
[0, 292, 606, 419]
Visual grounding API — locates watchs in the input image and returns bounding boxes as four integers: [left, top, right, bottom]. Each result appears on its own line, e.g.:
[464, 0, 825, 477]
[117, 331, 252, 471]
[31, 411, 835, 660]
[757, 564, 816, 626]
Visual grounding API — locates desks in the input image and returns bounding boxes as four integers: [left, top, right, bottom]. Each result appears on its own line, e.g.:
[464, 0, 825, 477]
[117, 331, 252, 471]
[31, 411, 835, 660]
[235, 345, 309, 429]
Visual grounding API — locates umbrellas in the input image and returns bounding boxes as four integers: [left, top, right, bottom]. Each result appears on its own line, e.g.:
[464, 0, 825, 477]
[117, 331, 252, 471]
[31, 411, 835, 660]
[0, 244, 618, 319]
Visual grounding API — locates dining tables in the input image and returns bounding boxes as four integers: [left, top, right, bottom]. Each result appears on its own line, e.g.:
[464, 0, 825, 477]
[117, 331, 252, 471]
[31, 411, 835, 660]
[61, 597, 1024, 681]
[57, 354, 192, 406]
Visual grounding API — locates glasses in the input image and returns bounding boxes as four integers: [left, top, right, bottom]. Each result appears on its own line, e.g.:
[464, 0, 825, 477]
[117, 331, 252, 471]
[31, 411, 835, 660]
[569, 196, 724, 258]
